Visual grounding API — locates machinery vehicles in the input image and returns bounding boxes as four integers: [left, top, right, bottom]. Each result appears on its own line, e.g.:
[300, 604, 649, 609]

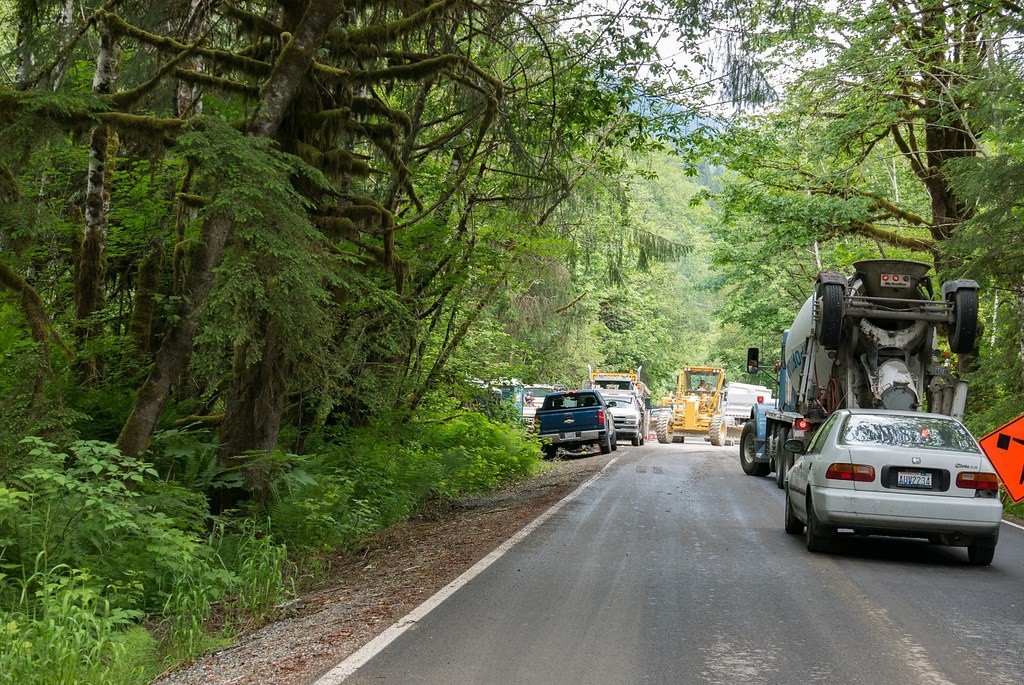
[657, 363, 781, 445]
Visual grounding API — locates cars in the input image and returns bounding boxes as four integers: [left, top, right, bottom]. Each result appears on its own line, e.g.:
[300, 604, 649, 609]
[783, 408, 1003, 567]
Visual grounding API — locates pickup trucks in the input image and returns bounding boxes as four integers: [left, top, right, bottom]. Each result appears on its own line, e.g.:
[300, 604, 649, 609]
[492, 366, 651, 459]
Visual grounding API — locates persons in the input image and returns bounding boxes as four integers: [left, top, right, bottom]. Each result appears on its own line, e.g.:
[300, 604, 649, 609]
[698, 379, 706, 389]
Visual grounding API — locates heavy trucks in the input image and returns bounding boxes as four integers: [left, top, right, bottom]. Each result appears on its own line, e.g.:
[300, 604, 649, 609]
[739, 258, 981, 486]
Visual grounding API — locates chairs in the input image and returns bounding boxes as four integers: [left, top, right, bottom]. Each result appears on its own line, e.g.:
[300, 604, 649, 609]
[583, 397, 595, 406]
[553, 399, 566, 409]
